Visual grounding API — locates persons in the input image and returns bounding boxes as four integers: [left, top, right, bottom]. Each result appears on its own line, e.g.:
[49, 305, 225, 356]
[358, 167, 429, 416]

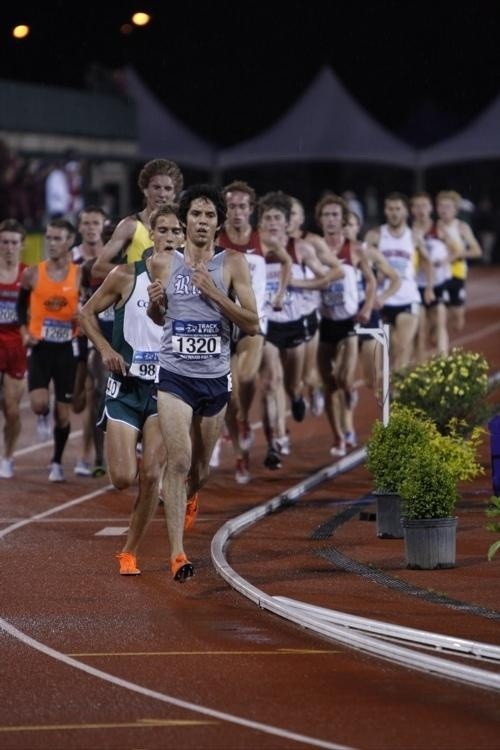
[365, 194, 420, 374]
[256, 192, 326, 471]
[147, 184, 260, 583]
[69, 210, 111, 478]
[16, 222, 84, 483]
[3, 149, 85, 220]
[213, 187, 292, 483]
[91, 158, 184, 280]
[0, 222, 33, 477]
[342, 196, 400, 410]
[286, 195, 346, 416]
[429, 191, 482, 355]
[311, 198, 377, 455]
[78, 217, 131, 477]
[76, 207, 182, 576]
[408, 195, 452, 360]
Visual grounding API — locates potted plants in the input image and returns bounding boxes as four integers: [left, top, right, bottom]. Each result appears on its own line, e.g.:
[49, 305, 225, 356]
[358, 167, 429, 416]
[363, 401, 437, 539]
[398, 432, 484, 569]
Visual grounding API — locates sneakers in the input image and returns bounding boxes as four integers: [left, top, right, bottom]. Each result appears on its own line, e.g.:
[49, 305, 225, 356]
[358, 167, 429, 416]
[290, 380, 306, 423]
[114, 551, 142, 576]
[236, 417, 255, 451]
[34, 413, 50, 441]
[73, 456, 92, 476]
[171, 552, 196, 584]
[1, 458, 15, 479]
[46, 461, 65, 482]
[234, 458, 253, 486]
[345, 432, 358, 448]
[330, 434, 345, 456]
[184, 492, 199, 532]
